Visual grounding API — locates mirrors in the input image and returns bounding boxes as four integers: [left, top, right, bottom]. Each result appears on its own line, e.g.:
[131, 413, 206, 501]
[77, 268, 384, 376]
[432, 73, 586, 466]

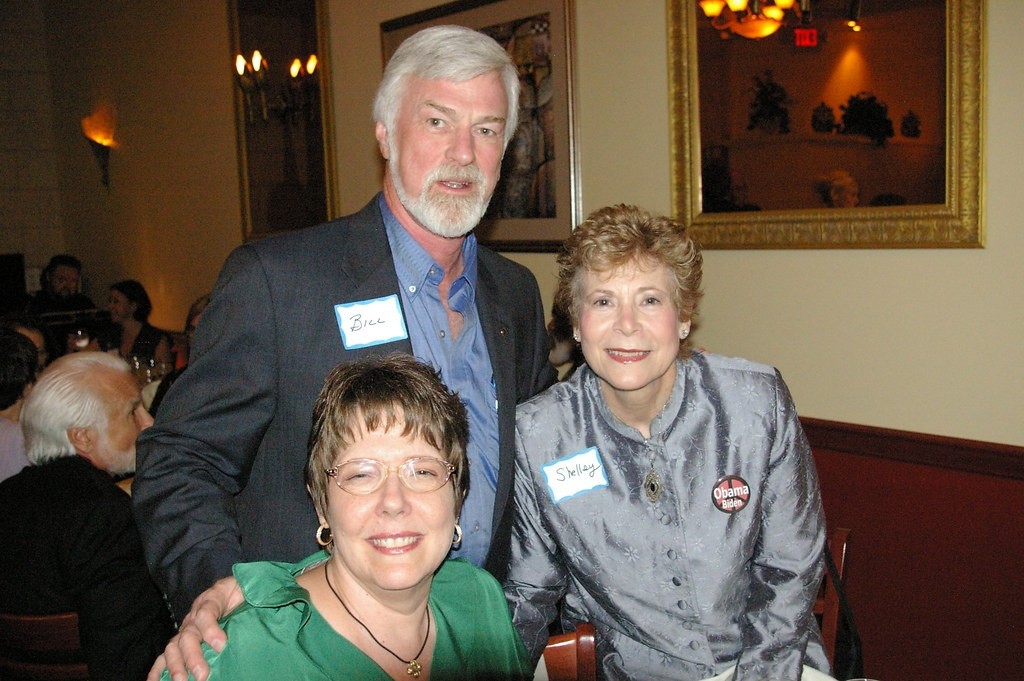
[225, 0, 338, 243]
[664, 0, 987, 252]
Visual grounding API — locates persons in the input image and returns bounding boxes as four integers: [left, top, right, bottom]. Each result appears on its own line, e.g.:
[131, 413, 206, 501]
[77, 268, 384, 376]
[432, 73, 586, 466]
[0, 254, 214, 681]
[502, 203, 844, 681]
[814, 167, 862, 208]
[132, 26, 559, 681]
[157, 352, 535, 681]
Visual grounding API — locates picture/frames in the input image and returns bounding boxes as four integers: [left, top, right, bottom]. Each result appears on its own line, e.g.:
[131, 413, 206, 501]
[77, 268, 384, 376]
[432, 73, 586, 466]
[379, 0, 584, 254]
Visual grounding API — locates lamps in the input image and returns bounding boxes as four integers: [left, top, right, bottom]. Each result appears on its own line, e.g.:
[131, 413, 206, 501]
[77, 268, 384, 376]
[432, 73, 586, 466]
[231, 47, 296, 125]
[699, 0, 795, 42]
[847, 0, 861, 28]
[281, 52, 319, 112]
[80, 116, 115, 194]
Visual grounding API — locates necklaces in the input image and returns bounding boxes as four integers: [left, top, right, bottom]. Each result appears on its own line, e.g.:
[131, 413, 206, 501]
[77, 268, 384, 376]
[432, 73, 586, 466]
[324, 558, 430, 678]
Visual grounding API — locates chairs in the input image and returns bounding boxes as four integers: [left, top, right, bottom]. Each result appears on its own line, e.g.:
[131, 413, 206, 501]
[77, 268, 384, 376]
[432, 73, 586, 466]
[0, 611, 91, 681]
[812, 525, 853, 664]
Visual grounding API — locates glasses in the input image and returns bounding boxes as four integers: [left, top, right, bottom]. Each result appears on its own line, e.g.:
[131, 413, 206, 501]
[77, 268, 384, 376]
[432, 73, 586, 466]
[323, 455, 456, 498]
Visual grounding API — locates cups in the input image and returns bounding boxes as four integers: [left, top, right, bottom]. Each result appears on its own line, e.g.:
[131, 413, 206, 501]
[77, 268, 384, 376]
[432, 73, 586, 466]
[71, 328, 89, 349]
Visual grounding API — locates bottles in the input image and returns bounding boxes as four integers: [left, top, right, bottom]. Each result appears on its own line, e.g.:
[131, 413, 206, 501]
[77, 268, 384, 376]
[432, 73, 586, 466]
[126, 353, 169, 389]
